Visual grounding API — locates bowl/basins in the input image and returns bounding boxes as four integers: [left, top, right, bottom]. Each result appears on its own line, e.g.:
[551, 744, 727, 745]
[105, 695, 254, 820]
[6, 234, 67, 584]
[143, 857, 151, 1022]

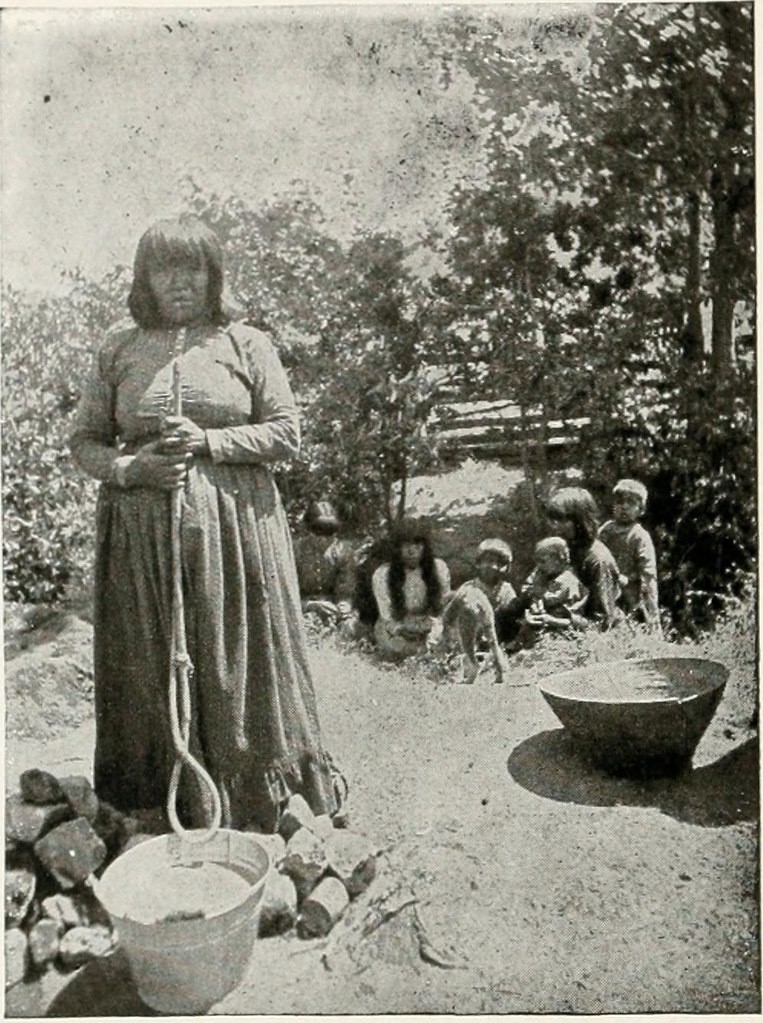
[537, 658, 731, 768]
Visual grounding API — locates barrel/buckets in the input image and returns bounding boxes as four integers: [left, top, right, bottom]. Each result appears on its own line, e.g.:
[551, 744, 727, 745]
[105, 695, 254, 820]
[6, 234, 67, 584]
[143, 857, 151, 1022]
[87, 827, 272, 1016]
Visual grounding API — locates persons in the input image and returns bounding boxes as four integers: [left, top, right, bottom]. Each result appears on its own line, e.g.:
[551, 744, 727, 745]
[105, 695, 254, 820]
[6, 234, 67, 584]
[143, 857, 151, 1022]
[354, 476, 661, 660]
[289, 496, 360, 636]
[72, 212, 341, 836]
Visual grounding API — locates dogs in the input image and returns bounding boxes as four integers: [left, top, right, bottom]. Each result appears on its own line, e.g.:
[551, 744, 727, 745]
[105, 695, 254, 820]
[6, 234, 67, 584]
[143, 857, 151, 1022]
[425, 584, 505, 686]
